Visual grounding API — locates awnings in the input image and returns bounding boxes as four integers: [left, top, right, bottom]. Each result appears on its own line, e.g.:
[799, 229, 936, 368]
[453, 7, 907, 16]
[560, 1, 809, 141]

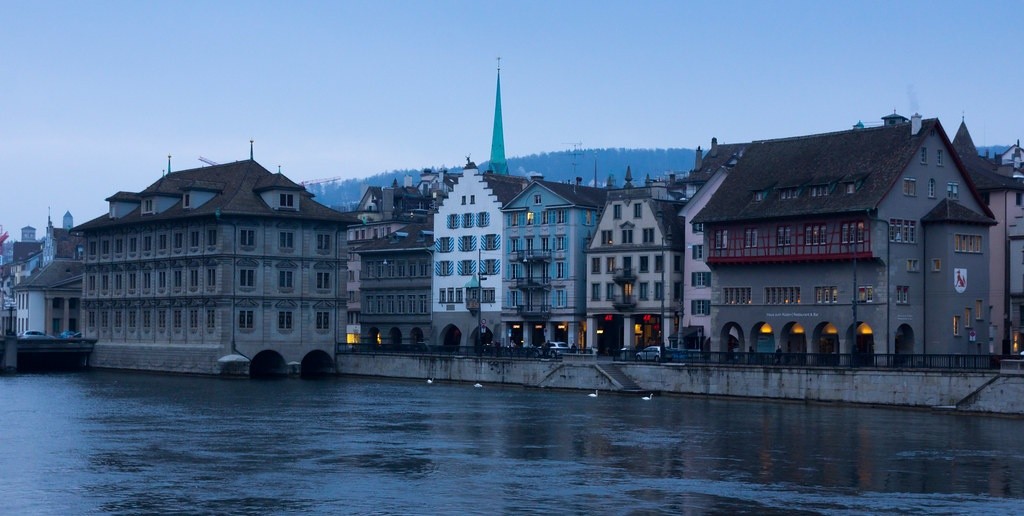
[668, 326, 704, 348]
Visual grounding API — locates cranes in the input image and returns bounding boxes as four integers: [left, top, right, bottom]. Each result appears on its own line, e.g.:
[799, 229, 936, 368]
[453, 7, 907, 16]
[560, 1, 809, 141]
[197, 155, 341, 187]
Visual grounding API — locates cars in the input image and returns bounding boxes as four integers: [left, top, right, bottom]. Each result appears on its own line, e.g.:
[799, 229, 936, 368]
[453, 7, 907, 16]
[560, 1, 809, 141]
[60, 331, 75, 337]
[635, 346, 665, 362]
[534, 342, 570, 357]
[18, 330, 54, 340]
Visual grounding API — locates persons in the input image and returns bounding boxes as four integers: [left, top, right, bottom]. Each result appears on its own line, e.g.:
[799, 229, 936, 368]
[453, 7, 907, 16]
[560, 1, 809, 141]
[749, 346, 754, 357]
[542, 340, 551, 355]
[571, 343, 577, 353]
[776, 345, 782, 361]
[510, 340, 516, 347]
